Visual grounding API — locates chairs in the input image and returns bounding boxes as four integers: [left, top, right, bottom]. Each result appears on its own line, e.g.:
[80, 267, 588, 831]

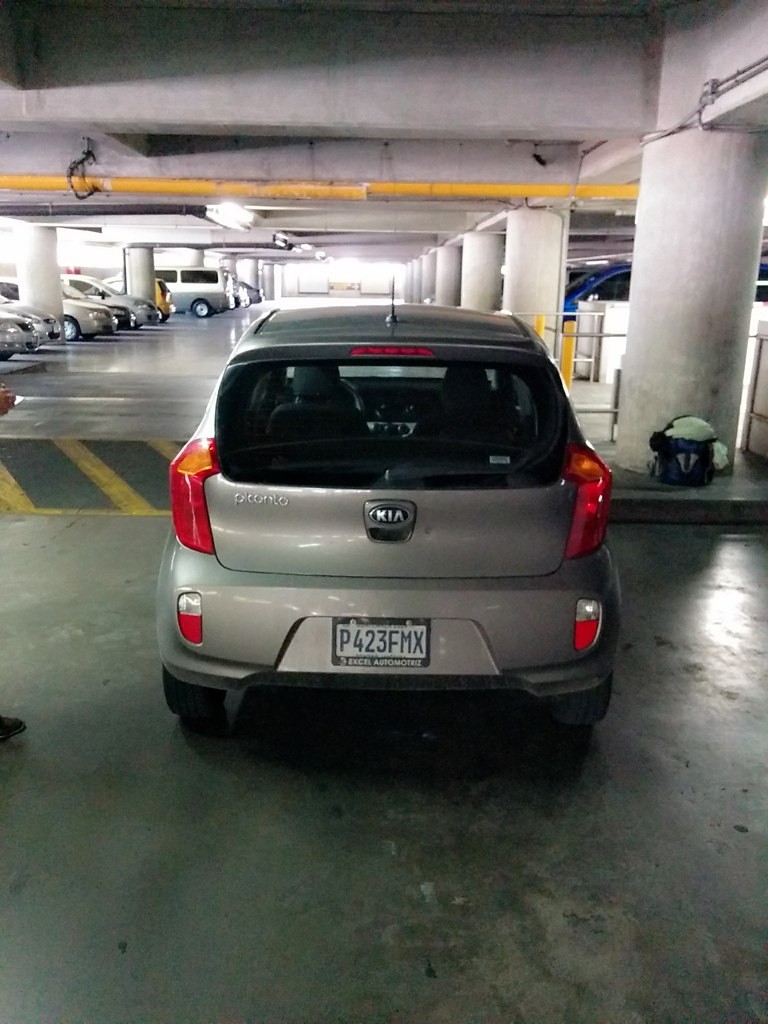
[408, 363, 513, 456]
[268, 365, 371, 451]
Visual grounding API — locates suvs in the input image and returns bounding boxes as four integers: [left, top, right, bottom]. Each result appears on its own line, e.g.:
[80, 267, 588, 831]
[154, 299, 624, 729]
[560, 262, 631, 321]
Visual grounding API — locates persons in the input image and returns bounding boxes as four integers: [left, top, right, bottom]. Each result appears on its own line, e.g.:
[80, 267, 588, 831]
[0, 381, 28, 745]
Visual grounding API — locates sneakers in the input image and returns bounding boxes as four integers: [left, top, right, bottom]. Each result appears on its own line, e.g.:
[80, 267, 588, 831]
[0, 715, 26, 741]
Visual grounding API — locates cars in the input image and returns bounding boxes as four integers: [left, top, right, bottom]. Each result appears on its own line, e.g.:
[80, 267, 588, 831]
[0, 270, 177, 358]
[230, 278, 267, 307]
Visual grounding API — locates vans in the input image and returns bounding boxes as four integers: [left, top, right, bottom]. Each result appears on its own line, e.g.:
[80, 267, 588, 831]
[109, 266, 232, 318]
